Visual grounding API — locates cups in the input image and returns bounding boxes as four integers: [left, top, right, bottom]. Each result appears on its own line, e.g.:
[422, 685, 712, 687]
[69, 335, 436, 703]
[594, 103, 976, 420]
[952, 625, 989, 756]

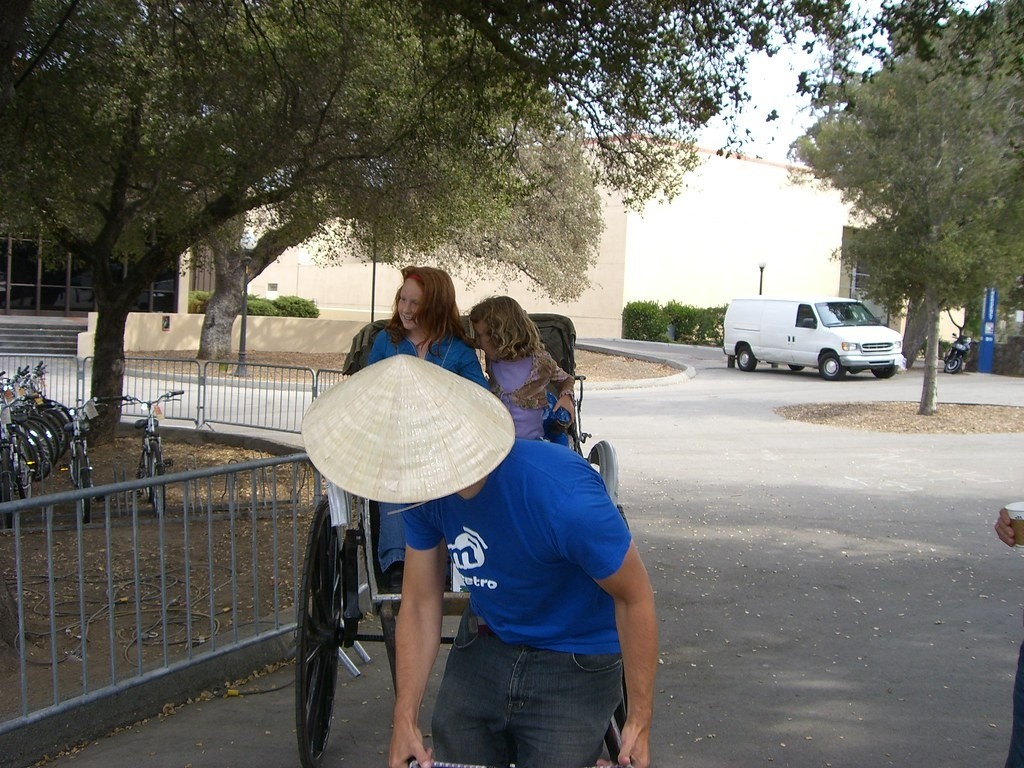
[1004, 502, 1024, 547]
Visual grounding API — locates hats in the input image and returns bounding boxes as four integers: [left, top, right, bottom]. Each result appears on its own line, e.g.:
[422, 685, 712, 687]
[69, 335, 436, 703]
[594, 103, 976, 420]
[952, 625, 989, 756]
[306, 355, 517, 504]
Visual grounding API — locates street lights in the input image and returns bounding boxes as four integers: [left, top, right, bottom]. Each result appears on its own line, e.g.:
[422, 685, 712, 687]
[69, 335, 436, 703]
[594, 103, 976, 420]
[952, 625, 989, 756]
[759, 260, 766, 296]
[232, 256, 252, 377]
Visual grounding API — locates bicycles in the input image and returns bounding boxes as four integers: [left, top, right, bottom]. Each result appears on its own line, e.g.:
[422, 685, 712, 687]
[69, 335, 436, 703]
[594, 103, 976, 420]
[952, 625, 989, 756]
[56, 395, 120, 524]
[0, 360, 75, 530]
[113, 389, 186, 521]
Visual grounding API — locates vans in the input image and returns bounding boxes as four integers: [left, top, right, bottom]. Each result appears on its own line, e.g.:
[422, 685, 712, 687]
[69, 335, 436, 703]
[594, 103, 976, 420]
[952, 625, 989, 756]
[723, 296, 903, 382]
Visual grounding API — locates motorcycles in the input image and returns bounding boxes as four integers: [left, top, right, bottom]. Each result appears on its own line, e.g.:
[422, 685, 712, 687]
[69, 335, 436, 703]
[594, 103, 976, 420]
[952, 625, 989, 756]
[943, 331, 977, 374]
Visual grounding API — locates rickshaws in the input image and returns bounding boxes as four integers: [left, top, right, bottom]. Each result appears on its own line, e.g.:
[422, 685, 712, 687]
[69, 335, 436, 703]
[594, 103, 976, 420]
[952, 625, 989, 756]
[291, 315, 640, 768]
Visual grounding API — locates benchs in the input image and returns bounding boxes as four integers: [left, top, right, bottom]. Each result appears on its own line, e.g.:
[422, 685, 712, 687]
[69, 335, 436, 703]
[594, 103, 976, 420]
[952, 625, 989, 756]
[340, 313, 585, 465]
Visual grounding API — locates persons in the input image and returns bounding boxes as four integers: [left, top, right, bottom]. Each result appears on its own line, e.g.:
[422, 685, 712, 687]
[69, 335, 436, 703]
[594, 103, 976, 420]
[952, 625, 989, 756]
[994, 497, 1024, 768]
[468, 295, 576, 442]
[366, 266, 489, 594]
[301, 353, 659, 768]
